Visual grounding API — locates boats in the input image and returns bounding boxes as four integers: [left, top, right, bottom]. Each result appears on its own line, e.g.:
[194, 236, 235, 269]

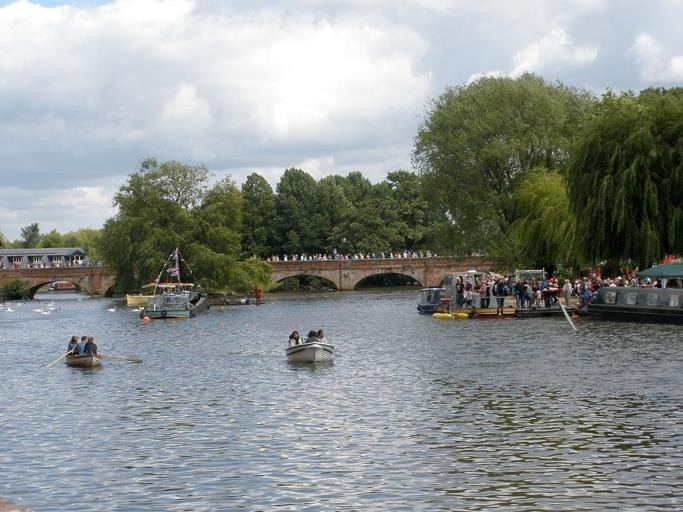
[417, 288, 447, 313]
[432, 311, 469, 319]
[66, 352, 102, 367]
[587, 286, 683, 323]
[285, 341, 334, 366]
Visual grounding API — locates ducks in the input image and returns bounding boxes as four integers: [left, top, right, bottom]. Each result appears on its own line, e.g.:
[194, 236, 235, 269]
[40, 310, 50, 315]
[32, 307, 44, 312]
[106, 307, 117, 312]
[49, 306, 57, 311]
[131, 305, 145, 312]
[45, 299, 55, 306]
[0, 300, 22, 313]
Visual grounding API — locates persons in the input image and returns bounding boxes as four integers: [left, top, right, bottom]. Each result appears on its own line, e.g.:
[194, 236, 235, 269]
[254, 285, 261, 303]
[83, 336, 97, 355]
[68, 335, 79, 355]
[266, 248, 436, 262]
[240, 295, 249, 304]
[39, 260, 63, 267]
[289, 329, 327, 348]
[455, 267, 682, 315]
[74, 336, 88, 355]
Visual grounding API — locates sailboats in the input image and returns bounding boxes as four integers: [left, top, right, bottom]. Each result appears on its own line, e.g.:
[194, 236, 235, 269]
[140, 246, 207, 319]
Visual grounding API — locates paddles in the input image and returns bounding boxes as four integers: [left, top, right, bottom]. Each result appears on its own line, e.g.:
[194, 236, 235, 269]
[97, 355, 142, 362]
